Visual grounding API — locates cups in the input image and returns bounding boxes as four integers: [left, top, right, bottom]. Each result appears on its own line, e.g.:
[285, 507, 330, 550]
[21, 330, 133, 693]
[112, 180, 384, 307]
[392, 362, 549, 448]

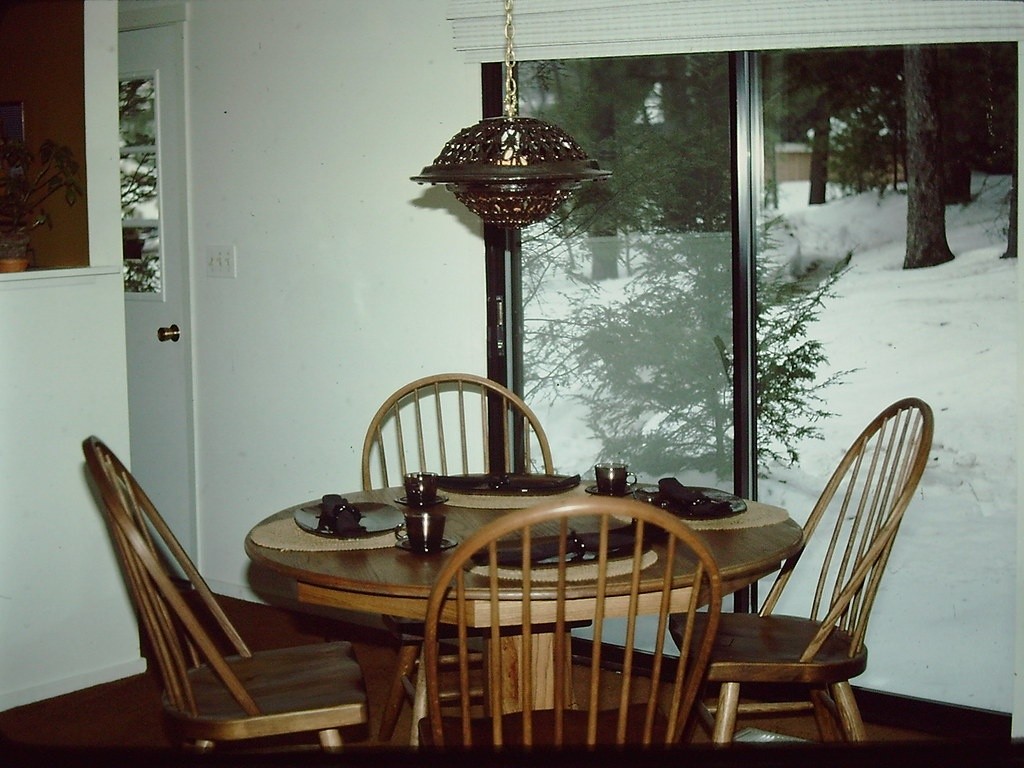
[403, 472, 436, 502]
[396, 513, 446, 547]
[596, 464, 636, 491]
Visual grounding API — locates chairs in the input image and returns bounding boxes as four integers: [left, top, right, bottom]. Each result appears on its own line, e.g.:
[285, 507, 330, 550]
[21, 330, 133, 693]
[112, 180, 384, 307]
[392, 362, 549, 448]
[362, 374, 593, 745]
[665, 397, 935, 750]
[82, 434, 369, 756]
[418, 497, 721, 752]
[244, 479, 804, 750]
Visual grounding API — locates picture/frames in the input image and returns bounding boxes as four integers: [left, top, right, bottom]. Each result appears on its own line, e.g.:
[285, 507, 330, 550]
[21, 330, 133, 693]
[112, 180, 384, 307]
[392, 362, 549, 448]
[0, 99, 25, 143]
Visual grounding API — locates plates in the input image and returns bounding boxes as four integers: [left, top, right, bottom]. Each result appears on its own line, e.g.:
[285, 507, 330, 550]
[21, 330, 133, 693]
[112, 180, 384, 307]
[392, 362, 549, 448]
[395, 538, 457, 552]
[295, 502, 403, 534]
[633, 485, 747, 519]
[395, 496, 449, 505]
[586, 484, 642, 496]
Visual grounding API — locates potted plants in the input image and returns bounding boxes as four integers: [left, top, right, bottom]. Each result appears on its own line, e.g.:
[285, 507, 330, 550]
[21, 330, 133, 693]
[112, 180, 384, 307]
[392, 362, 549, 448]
[0, 135, 86, 274]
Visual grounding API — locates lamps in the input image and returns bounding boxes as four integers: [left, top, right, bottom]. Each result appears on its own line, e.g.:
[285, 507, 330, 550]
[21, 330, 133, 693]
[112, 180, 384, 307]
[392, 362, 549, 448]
[408, 0, 615, 230]
